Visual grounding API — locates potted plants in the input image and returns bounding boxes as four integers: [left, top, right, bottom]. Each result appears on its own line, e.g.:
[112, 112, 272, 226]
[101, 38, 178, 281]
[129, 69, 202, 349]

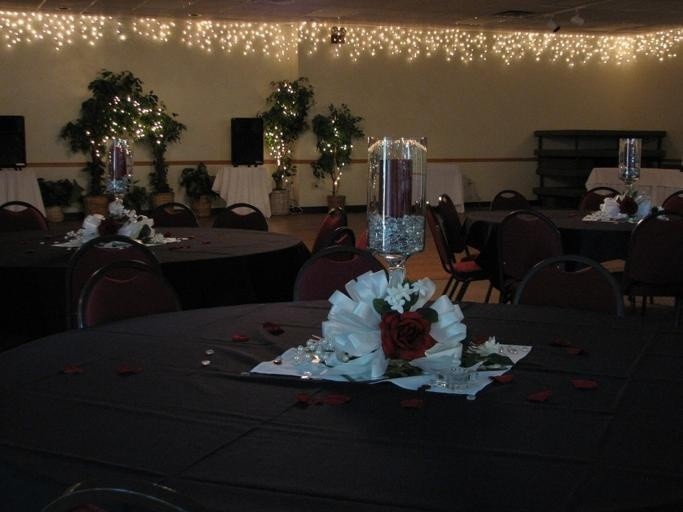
[310, 103, 365, 210]
[36, 68, 221, 223]
[256, 78, 316, 216]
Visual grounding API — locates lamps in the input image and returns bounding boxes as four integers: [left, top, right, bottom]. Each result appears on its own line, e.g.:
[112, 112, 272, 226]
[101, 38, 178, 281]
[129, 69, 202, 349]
[543, 0, 607, 33]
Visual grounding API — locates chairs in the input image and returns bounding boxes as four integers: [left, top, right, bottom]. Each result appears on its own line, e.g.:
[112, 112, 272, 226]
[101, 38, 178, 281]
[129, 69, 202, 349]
[41, 478, 211, 512]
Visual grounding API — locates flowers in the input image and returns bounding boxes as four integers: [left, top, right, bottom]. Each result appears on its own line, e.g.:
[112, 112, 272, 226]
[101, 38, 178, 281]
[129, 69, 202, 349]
[321, 271, 468, 379]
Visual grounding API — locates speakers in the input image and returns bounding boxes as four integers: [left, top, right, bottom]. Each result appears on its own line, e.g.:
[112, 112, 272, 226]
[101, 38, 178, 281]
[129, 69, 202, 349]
[0, 115, 28, 168]
[230, 117, 264, 165]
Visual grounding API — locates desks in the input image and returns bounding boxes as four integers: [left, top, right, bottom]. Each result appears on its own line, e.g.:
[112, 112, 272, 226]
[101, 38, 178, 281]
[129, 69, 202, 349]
[0, 168, 46, 218]
[211, 167, 276, 218]
[0, 299, 683, 512]
